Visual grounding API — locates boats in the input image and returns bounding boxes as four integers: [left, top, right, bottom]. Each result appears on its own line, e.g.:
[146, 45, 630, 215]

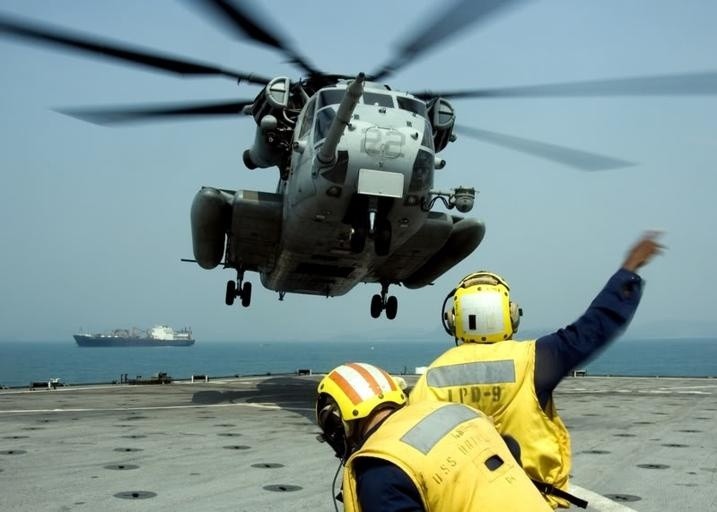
[70, 324, 200, 351]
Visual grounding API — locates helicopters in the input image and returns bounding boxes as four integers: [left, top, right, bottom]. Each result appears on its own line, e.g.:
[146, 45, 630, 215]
[0, 0, 717, 321]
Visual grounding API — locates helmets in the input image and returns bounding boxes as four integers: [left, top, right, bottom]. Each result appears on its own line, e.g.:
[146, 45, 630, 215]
[314, 361, 409, 466]
[452, 271, 513, 344]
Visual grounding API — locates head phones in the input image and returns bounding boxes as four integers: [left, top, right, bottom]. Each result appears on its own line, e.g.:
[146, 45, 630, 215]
[319, 402, 354, 447]
[441, 272, 523, 334]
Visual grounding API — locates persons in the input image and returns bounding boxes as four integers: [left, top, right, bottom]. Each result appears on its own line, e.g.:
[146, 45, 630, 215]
[405, 227, 671, 512]
[314, 359, 559, 512]
